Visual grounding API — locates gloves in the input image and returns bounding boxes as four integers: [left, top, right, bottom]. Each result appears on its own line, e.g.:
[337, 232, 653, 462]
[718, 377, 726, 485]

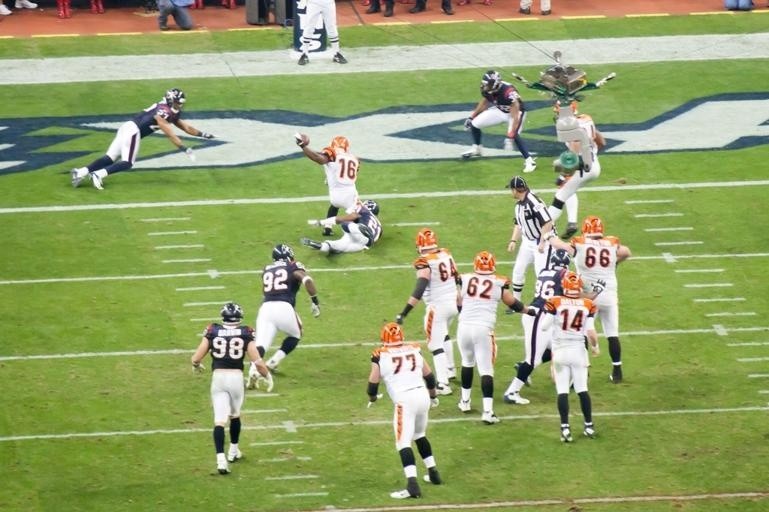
[264, 372, 276, 391]
[294, 133, 305, 148]
[190, 362, 205, 376]
[306, 220, 320, 227]
[178, 144, 192, 154]
[310, 296, 325, 317]
[203, 132, 221, 139]
[430, 397, 438, 409]
[590, 280, 607, 293]
[463, 116, 472, 129]
[502, 137, 513, 154]
[592, 345, 599, 358]
[366, 394, 382, 407]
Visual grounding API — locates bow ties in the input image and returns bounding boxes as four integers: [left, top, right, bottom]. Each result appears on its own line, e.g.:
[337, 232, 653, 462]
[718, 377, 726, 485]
[0, 2, 105, 20]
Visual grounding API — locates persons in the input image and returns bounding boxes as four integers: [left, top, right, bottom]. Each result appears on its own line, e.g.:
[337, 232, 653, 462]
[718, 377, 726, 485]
[408, 2, 492, 16]
[293, 134, 383, 257]
[153, 0, 196, 34]
[365, 1, 395, 17]
[70, 89, 221, 191]
[462, 71, 536, 174]
[0, 0, 38, 17]
[724, 0, 754, 13]
[392, 233, 537, 423]
[520, 1, 552, 15]
[294, 1, 349, 65]
[245, 243, 322, 390]
[546, 100, 605, 240]
[503, 176, 634, 435]
[190, 301, 275, 474]
[366, 320, 442, 498]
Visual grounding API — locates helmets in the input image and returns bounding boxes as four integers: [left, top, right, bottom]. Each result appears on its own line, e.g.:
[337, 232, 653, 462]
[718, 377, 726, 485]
[332, 135, 380, 215]
[164, 87, 187, 106]
[480, 70, 502, 95]
[271, 242, 292, 263]
[381, 321, 405, 346]
[221, 300, 244, 324]
[416, 215, 604, 298]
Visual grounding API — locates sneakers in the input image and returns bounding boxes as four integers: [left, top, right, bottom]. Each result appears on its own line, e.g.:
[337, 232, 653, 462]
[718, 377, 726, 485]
[609, 369, 622, 383]
[332, 52, 345, 64]
[69, 166, 81, 189]
[462, 144, 483, 158]
[87, 172, 102, 189]
[422, 473, 443, 485]
[245, 372, 257, 390]
[504, 306, 517, 315]
[582, 421, 595, 437]
[523, 157, 537, 173]
[299, 225, 372, 251]
[560, 425, 573, 443]
[389, 488, 422, 499]
[434, 360, 533, 425]
[297, 52, 309, 66]
[360, 0, 552, 17]
[216, 442, 244, 475]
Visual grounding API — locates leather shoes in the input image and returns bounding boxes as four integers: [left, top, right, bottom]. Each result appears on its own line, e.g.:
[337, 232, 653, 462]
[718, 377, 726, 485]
[158, 20, 168, 31]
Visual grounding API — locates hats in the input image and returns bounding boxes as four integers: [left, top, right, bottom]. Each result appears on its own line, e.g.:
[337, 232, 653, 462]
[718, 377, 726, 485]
[504, 177, 527, 189]
